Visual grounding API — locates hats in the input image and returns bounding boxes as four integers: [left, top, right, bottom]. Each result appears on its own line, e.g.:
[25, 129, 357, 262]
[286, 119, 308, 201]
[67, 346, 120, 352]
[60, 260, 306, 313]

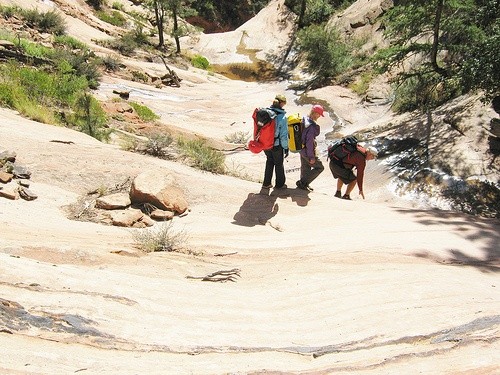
[313, 105, 324, 117]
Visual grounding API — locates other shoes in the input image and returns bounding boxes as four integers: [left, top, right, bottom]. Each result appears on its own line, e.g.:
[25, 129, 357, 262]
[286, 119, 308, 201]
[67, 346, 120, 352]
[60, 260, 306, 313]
[308, 186, 313, 191]
[262, 184, 273, 188]
[342, 194, 351, 200]
[280, 184, 287, 188]
[335, 192, 341, 197]
[296, 181, 309, 191]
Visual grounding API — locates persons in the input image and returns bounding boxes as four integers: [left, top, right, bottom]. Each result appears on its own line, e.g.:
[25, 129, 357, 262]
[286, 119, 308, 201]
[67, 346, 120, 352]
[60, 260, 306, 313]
[262, 95, 289, 189]
[329, 144, 378, 200]
[296, 105, 324, 192]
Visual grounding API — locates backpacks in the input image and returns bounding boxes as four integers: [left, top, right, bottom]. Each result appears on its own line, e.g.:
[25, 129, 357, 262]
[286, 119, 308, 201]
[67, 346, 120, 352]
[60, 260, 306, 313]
[252, 107, 281, 150]
[287, 113, 306, 153]
[328, 136, 366, 170]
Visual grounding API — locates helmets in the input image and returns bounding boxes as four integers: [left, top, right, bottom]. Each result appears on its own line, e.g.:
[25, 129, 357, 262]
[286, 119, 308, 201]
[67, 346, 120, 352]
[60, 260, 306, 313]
[249, 141, 264, 154]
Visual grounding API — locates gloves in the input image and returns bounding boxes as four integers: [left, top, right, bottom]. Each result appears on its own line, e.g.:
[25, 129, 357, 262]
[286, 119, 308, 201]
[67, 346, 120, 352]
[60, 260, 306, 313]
[284, 148, 288, 158]
